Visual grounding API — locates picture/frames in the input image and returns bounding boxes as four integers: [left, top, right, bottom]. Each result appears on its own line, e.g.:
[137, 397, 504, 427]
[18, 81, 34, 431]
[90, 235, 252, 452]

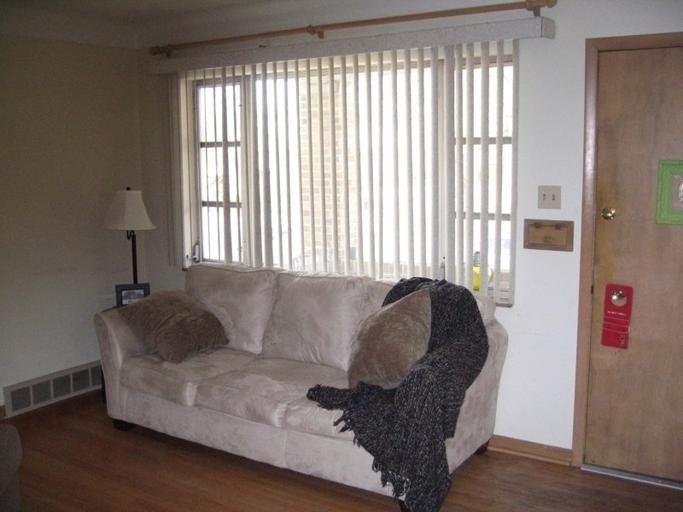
[115, 283, 150, 308]
[657, 160, 682, 223]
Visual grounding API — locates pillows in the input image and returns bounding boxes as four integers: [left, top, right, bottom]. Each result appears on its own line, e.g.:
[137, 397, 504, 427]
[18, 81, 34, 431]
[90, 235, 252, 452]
[350, 287, 433, 391]
[121, 286, 227, 365]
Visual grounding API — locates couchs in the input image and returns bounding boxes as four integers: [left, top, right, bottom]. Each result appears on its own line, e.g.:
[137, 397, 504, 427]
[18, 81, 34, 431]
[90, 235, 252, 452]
[93, 259, 510, 510]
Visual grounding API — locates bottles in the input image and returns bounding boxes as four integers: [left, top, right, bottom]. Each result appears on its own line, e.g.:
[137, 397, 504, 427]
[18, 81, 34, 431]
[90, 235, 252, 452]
[473, 251, 481, 291]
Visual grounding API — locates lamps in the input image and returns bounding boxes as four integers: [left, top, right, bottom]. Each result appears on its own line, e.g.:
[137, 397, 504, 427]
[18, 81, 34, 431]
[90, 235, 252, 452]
[99, 185, 157, 284]
